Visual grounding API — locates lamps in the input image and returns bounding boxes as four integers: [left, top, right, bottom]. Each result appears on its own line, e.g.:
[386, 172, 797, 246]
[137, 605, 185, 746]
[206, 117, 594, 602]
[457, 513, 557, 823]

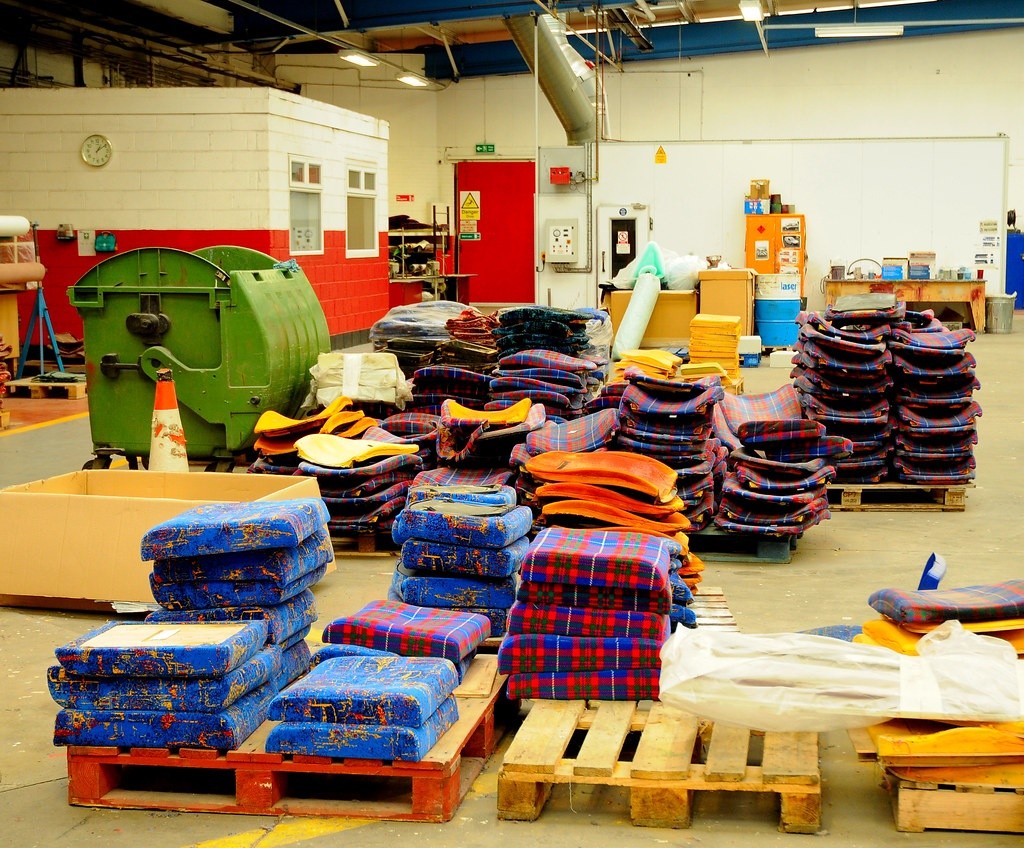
[814, 25, 904, 39]
[339, 50, 380, 67]
[395, 71, 429, 89]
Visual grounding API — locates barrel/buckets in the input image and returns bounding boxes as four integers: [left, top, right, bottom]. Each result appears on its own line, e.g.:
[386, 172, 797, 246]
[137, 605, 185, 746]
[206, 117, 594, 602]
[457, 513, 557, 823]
[744, 198, 770, 214]
[751, 179, 769, 199]
[754, 274, 802, 347]
[831, 265, 845, 279]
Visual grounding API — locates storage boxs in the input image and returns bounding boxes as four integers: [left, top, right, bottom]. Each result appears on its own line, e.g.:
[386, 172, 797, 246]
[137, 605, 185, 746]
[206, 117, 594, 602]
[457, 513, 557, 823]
[697, 267, 758, 336]
[755, 322, 800, 345]
[755, 298, 801, 324]
[738, 336, 761, 353]
[755, 274, 802, 301]
[0, 469, 338, 613]
[610, 290, 699, 350]
[744, 354, 759, 366]
[770, 351, 798, 368]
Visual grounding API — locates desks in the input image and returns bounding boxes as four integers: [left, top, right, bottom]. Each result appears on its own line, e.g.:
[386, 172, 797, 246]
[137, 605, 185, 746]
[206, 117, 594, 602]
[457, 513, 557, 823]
[824, 277, 989, 333]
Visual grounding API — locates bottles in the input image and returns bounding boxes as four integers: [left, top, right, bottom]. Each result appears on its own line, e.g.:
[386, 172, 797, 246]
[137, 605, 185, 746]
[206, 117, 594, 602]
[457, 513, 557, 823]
[854, 266, 874, 278]
[938, 265, 956, 278]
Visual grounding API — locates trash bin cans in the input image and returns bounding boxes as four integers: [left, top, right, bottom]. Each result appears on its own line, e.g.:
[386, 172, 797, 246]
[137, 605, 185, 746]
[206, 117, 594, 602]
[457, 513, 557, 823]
[986, 297, 1015, 334]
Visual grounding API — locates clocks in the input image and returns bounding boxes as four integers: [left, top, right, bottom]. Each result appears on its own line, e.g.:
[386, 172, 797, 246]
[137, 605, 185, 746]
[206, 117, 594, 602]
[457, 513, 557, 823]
[80, 134, 111, 167]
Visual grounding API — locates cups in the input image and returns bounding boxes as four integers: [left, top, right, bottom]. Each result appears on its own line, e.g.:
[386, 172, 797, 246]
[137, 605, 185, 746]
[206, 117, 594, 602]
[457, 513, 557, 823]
[958, 270, 984, 279]
[770, 194, 795, 214]
[706, 255, 721, 268]
